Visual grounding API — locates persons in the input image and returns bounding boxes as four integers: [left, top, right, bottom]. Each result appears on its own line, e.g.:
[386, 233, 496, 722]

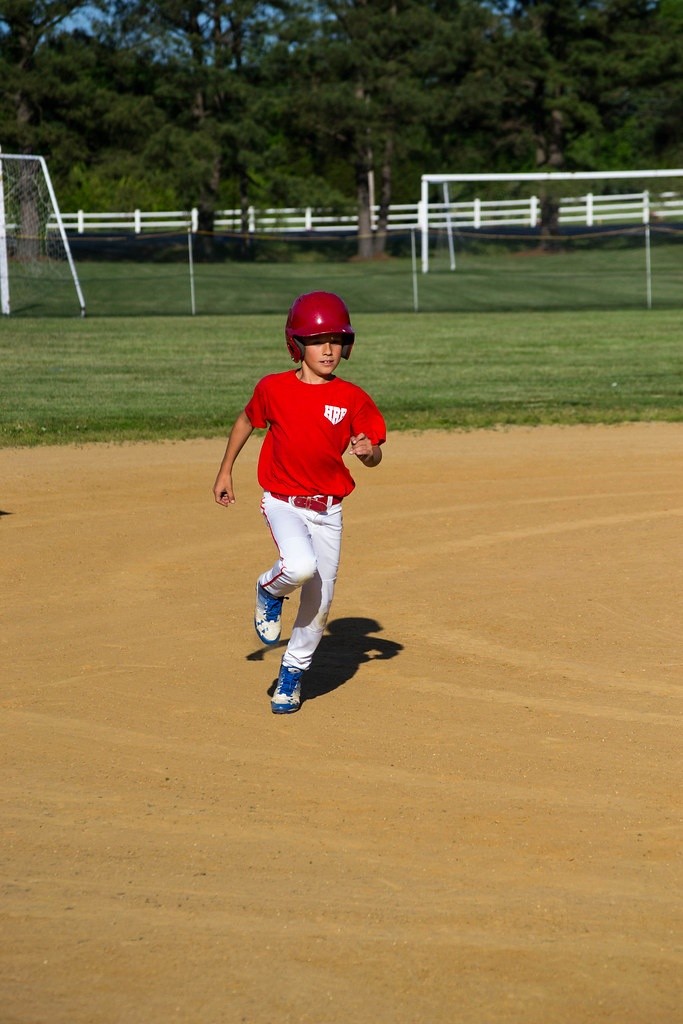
[212, 290, 387, 714]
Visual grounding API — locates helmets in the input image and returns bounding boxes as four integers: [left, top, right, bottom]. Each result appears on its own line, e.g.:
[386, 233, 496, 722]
[285, 291, 355, 363]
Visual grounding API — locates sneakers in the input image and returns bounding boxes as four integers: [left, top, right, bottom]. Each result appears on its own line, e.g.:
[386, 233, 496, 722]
[271, 664, 306, 714]
[255, 576, 289, 644]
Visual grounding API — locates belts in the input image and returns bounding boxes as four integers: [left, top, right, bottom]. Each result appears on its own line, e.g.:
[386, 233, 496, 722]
[271, 492, 341, 511]
